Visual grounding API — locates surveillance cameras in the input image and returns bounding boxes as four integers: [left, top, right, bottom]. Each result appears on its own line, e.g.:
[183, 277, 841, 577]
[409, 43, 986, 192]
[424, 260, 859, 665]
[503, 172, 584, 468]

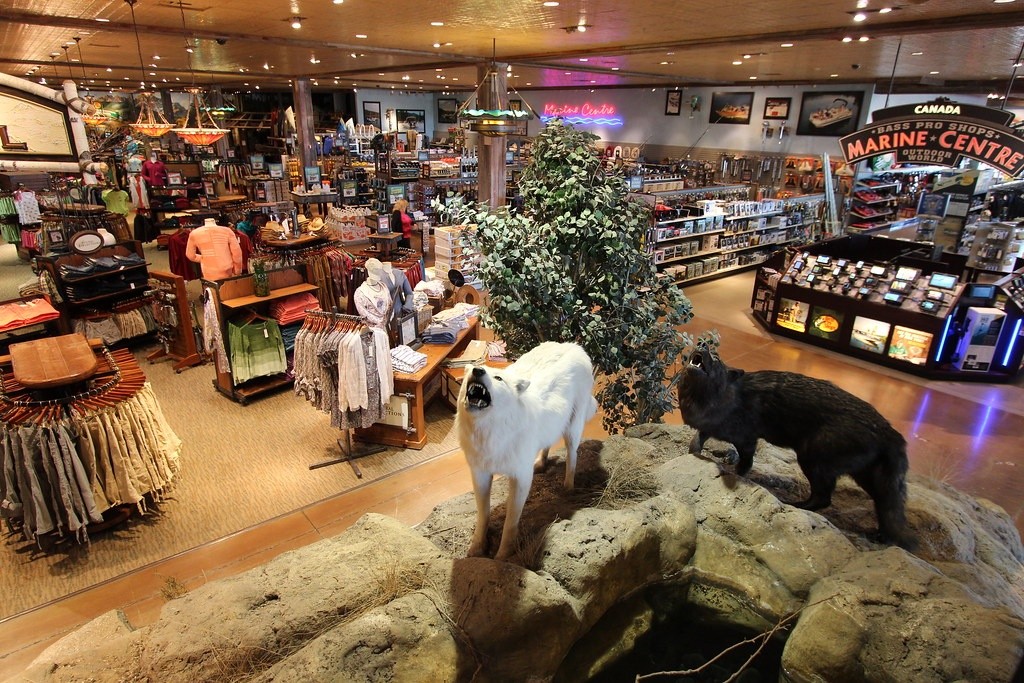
[215, 38, 226, 45]
[852, 64, 860, 69]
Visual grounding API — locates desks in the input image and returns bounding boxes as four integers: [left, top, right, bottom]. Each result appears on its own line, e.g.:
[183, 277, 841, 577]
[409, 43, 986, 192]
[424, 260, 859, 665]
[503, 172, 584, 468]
[352, 315, 483, 450]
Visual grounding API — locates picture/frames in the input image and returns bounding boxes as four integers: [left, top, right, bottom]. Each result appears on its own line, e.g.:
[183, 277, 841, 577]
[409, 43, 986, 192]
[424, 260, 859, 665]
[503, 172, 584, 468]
[665, 89, 865, 137]
[362, 98, 522, 134]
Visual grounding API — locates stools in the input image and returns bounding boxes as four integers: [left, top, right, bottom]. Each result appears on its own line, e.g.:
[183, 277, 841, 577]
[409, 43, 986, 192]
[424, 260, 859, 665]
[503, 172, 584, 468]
[436, 354, 515, 414]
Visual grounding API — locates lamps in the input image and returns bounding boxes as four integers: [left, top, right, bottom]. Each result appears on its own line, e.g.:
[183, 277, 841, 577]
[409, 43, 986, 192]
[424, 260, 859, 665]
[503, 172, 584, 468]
[454, 38, 540, 137]
[25, 0, 231, 146]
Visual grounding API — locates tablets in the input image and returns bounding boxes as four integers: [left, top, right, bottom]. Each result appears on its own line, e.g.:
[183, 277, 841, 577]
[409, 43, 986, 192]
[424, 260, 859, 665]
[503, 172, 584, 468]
[789, 251, 959, 313]
[965, 283, 997, 302]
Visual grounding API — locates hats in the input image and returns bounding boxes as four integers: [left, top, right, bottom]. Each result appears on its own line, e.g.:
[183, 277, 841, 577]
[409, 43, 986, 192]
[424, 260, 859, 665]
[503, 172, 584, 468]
[454, 284, 480, 305]
[365, 257, 383, 281]
[289, 213, 312, 233]
[260, 220, 285, 241]
[307, 217, 329, 234]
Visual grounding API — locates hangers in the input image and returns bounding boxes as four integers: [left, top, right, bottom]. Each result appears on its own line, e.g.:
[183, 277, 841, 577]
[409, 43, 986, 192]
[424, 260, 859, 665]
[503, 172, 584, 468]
[0, 181, 424, 428]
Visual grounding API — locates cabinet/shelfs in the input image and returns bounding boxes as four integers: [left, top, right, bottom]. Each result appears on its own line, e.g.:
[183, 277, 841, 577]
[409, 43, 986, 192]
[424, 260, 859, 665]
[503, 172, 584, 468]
[595, 151, 993, 286]
[204, 123, 537, 479]
